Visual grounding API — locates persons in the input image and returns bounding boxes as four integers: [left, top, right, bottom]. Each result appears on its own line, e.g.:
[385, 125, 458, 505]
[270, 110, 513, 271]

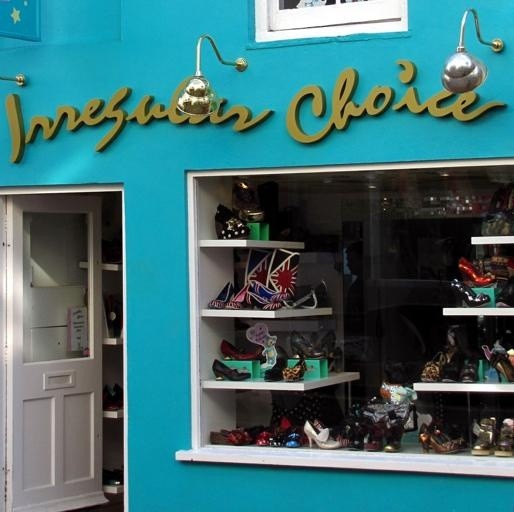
[345, 233, 465, 380]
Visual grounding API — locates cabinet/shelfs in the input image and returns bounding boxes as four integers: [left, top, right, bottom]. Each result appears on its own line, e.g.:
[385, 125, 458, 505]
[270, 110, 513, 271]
[198, 239, 360, 392]
[76, 261, 123, 493]
[413, 235, 514, 395]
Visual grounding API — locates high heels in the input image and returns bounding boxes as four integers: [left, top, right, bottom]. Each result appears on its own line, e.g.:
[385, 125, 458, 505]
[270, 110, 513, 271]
[457, 255, 495, 287]
[219, 339, 257, 360]
[212, 181, 266, 241]
[495, 274, 514, 307]
[282, 359, 307, 382]
[209, 397, 403, 453]
[289, 331, 345, 373]
[449, 278, 490, 308]
[263, 357, 288, 382]
[428, 422, 469, 452]
[251, 280, 289, 303]
[419, 323, 514, 383]
[212, 359, 252, 381]
[417, 424, 462, 454]
[101, 235, 123, 487]
[207, 281, 234, 309]
[224, 283, 252, 310]
[483, 181, 514, 224]
[244, 288, 283, 310]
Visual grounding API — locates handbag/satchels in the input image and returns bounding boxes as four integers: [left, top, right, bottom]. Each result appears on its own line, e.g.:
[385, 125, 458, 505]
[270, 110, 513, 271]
[267, 204, 306, 242]
[292, 278, 328, 310]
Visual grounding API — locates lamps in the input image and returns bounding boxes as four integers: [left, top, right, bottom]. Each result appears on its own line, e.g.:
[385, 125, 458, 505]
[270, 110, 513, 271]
[176, 32, 247, 117]
[441, 9, 503, 94]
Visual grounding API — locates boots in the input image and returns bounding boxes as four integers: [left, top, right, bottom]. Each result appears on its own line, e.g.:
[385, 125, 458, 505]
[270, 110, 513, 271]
[471, 415, 497, 456]
[492, 417, 514, 457]
